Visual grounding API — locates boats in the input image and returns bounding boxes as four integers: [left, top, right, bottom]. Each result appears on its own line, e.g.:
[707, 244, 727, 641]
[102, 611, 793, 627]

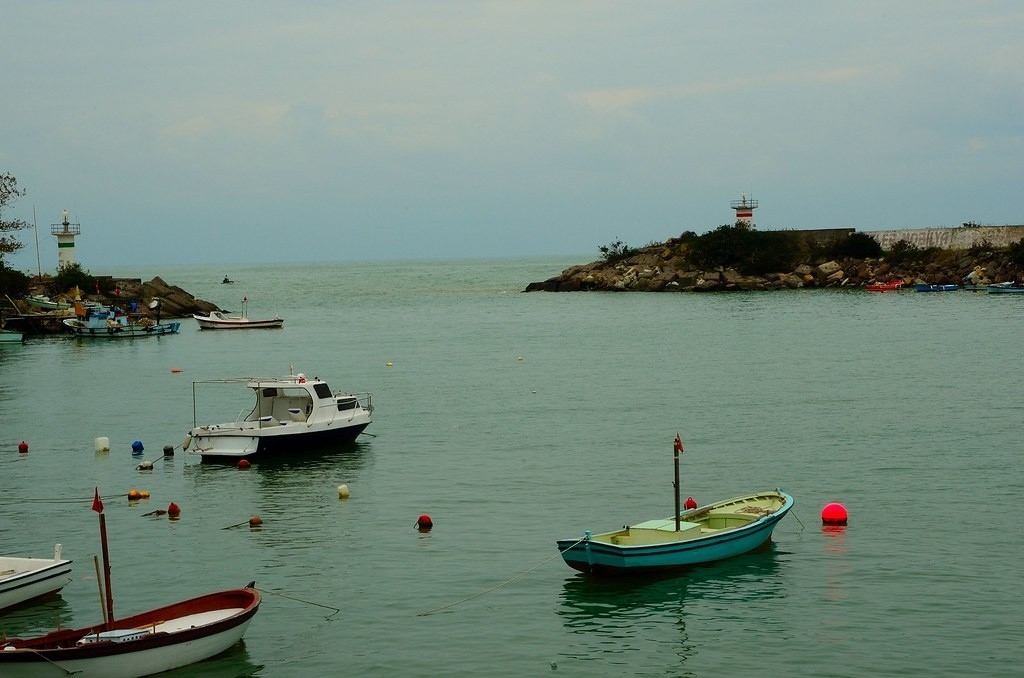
[913, 282, 957, 292]
[0, 544, 73, 615]
[987, 282, 1024, 295]
[63, 313, 180, 336]
[0, 485, 262, 678]
[221, 276, 234, 284]
[190, 365, 374, 465]
[0, 330, 24, 344]
[964, 283, 989, 289]
[866, 282, 904, 291]
[557, 434, 794, 579]
[25, 295, 111, 313]
[192, 297, 284, 328]
